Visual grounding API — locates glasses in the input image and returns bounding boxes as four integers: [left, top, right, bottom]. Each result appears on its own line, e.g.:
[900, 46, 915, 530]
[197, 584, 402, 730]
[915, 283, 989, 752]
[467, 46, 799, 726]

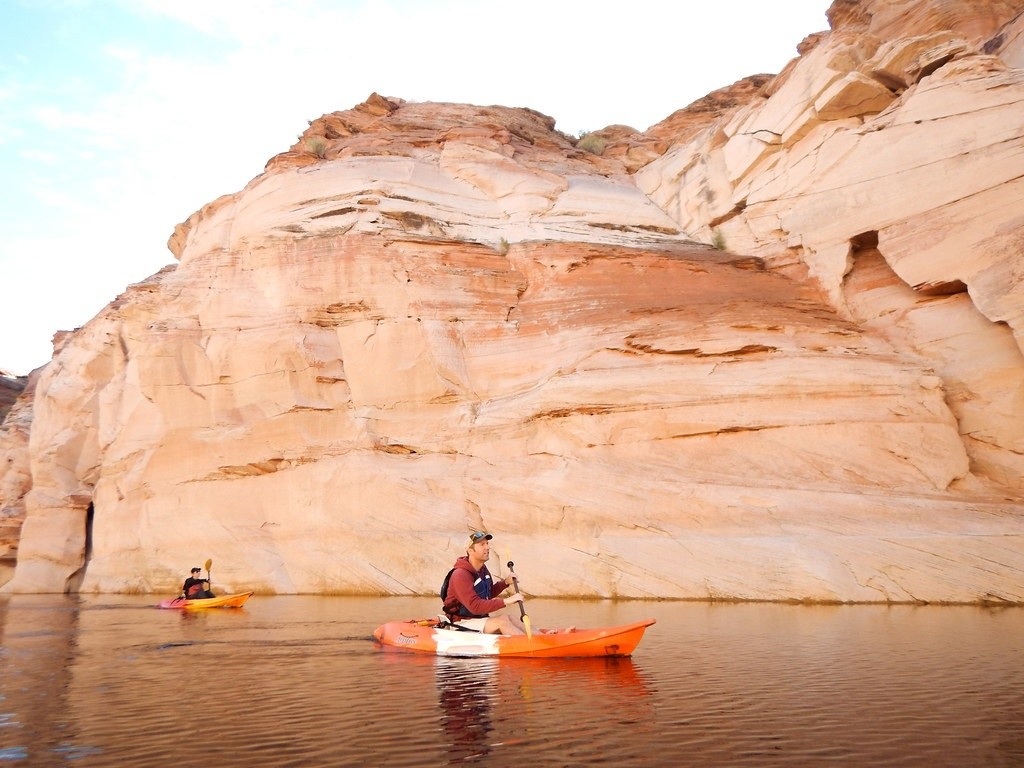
[195, 570, 200, 573]
[470, 532, 486, 543]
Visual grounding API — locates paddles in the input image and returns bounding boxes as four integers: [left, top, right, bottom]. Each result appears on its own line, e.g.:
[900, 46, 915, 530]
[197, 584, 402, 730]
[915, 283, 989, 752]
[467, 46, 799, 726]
[504, 544, 533, 655]
[205, 559, 212, 590]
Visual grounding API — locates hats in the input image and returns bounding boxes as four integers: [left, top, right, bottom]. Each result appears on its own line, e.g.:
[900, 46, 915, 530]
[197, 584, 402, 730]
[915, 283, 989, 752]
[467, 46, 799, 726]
[464, 532, 492, 551]
[191, 568, 201, 573]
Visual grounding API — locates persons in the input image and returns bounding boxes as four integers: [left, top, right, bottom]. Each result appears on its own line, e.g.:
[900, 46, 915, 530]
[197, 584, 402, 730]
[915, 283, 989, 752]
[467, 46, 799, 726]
[440, 532, 576, 635]
[181, 568, 216, 600]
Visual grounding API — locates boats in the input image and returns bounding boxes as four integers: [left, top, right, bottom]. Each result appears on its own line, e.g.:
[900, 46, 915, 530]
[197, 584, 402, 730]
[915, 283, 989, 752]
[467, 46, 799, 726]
[372, 617, 657, 657]
[157, 591, 254, 610]
